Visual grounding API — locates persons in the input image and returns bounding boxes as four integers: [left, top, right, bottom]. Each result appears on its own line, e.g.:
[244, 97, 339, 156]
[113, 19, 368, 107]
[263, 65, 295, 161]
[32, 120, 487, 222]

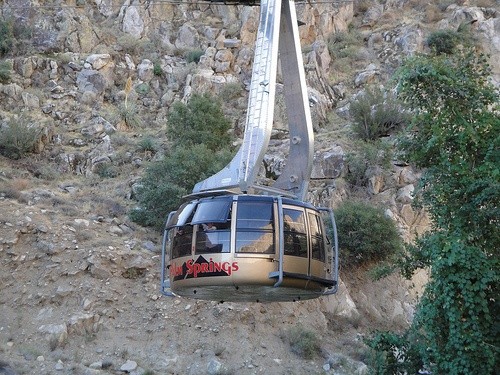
[201, 220, 219, 254]
